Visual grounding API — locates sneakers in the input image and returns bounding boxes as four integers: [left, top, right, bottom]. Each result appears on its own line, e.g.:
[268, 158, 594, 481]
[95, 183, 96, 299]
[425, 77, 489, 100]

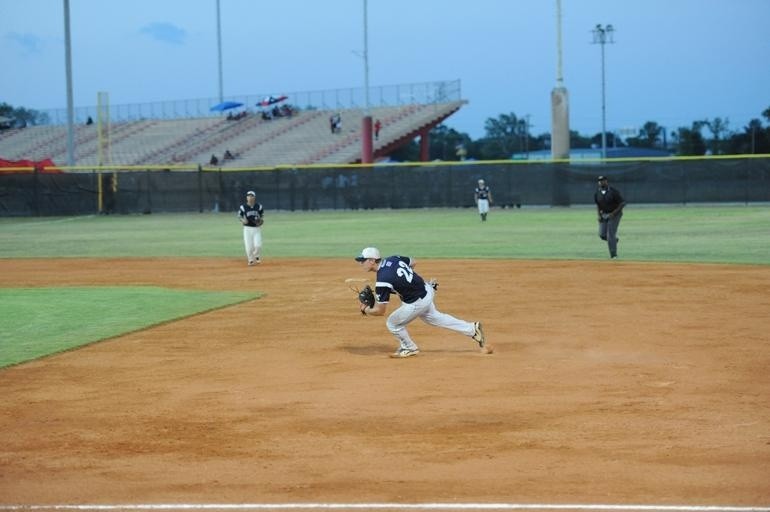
[248, 257, 260, 265]
[472, 322, 484, 347]
[391, 348, 419, 358]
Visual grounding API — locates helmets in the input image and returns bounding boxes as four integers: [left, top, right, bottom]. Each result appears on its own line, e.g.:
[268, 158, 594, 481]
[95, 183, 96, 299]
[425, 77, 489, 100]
[246, 191, 255, 196]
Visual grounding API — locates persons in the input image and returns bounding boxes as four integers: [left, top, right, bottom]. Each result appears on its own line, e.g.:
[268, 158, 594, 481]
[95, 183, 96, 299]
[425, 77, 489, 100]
[237, 190, 265, 266]
[329, 115, 342, 134]
[209, 150, 233, 166]
[594, 175, 627, 261]
[210, 103, 291, 121]
[375, 118, 381, 139]
[474, 179, 493, 220]
[354, 248, 484, 359]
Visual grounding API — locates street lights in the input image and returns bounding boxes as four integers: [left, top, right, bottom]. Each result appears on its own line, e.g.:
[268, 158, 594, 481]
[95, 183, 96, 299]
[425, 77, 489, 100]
[593, 23, 614, 159]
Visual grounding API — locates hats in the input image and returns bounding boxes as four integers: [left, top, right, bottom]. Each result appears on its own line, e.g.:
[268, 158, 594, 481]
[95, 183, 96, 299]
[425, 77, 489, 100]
[355, 247, 381, 259]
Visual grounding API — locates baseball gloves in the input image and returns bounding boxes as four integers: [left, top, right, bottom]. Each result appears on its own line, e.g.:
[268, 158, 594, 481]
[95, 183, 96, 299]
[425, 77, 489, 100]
[255, 218, 263, 226]
[359, 285, 375, 309]
[600, 214, 609, 223]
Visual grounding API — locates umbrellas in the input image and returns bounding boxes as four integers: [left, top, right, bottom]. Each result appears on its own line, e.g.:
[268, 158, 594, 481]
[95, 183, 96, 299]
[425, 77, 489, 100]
[208, 101, 244, 112]
[255, 95, 288, 107]
